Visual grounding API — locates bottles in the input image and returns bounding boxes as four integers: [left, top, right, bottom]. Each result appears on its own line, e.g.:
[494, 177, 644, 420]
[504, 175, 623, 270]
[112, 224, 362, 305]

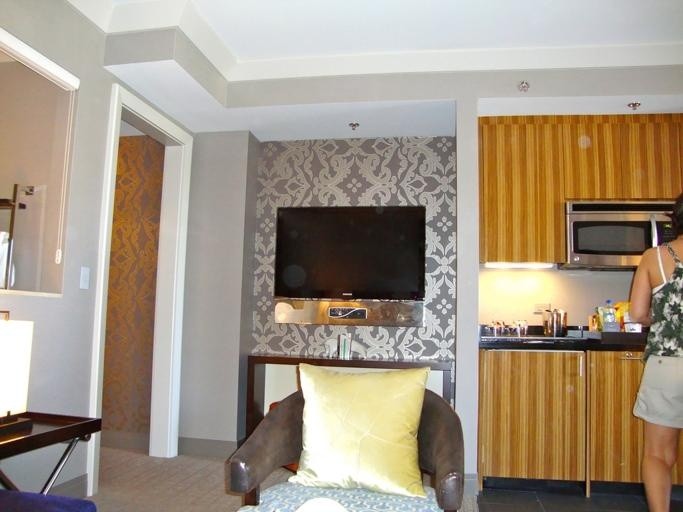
[605, 300, 616, 332]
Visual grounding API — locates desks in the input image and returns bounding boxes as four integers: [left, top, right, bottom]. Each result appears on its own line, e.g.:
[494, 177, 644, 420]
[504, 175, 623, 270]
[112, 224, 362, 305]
[0, 411, 101, 495]
[246, 356, 451, 473]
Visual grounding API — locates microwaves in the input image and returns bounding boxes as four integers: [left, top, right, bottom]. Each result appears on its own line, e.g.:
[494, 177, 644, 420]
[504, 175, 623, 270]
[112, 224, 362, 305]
[564, 201, 676, 271]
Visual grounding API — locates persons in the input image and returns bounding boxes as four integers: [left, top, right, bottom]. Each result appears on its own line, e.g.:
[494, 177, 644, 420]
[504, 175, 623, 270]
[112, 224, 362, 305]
[629, 190, 683, 511]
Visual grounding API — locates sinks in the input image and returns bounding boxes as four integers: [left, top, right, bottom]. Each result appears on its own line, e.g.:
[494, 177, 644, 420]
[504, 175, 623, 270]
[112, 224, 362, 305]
[480, 335, 583, 344]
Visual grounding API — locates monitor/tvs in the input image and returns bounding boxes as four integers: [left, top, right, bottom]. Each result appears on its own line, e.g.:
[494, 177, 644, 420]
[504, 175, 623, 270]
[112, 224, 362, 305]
[274, 205, 425, 299]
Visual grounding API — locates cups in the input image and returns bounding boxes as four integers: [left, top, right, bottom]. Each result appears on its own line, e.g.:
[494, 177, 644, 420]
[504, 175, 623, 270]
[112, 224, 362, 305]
[515, 319, 528, 336]
[494, 320, 504, 337]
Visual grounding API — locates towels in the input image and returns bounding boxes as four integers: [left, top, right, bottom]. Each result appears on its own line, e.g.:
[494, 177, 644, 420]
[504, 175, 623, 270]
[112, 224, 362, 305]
[0, 231, 15, 289]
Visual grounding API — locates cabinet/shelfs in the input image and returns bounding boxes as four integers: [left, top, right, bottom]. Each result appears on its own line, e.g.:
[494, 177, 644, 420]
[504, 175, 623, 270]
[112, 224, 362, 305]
[566, 122, 682, 200]
[0, 27, 81, 298]
[480, 123, 566, 263]
[586, 351, 678, 485]
[478, 349, 587, 482]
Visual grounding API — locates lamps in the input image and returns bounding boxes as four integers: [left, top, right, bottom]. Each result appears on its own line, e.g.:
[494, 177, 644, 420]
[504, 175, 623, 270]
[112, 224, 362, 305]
[0, 320, 34, 436]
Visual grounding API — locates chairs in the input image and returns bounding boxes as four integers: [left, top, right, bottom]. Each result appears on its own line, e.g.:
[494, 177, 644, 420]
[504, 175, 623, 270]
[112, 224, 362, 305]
[225, 389, 464, 512]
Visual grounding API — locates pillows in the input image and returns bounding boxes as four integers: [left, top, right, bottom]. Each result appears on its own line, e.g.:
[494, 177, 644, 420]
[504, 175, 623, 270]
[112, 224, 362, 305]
[288, 362, 431, 498]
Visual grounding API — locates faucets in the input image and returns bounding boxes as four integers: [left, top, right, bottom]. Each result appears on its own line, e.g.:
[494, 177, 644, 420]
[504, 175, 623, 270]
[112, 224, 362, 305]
[546, 307, 559, 338]
